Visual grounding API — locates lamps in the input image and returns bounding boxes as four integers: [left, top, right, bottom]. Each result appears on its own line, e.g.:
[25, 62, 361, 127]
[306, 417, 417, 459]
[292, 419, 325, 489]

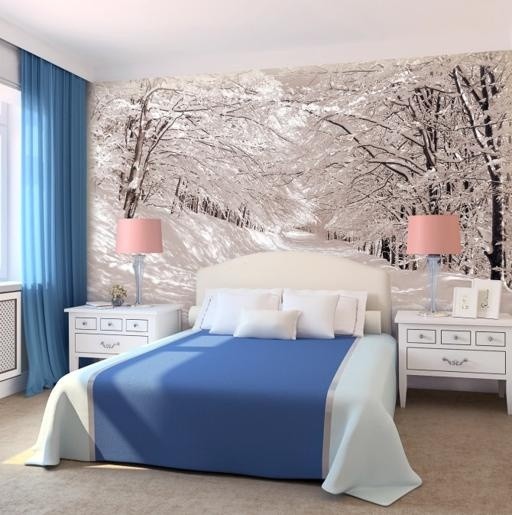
[113, 218, 163, 307]
[405, 215, 460, 317]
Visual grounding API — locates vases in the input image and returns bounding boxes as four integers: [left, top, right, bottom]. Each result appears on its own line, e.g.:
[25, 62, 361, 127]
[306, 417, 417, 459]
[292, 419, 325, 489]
[111, 298, 123, 306]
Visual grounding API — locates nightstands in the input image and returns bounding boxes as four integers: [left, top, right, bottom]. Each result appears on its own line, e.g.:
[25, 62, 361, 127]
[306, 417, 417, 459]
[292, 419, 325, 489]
[393, 308, 512, 417]
[64, 303, 182, 373]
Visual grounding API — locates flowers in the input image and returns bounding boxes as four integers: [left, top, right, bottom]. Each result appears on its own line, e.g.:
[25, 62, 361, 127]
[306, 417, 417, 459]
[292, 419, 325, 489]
[109, 285, 127, 302]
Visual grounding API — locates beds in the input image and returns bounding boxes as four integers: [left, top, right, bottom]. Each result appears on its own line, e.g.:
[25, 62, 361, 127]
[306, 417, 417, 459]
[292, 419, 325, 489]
[63, 249, 397, 481]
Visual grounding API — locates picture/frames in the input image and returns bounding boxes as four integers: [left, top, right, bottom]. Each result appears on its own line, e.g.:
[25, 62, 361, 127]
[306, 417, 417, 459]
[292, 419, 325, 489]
[452, 279, 501, 319]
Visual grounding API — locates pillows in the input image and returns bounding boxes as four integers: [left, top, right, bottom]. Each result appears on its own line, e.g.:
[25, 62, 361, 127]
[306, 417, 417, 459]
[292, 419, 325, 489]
[191, 287, 381, 343]
